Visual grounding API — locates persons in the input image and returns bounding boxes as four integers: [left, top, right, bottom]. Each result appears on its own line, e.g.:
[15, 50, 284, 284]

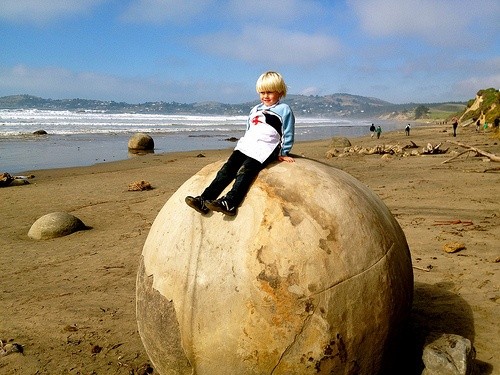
[452, 120, 458, 137]
[376, 126, 382, 139]
[405, 124, 410, 136]
[186, 72, 295, 217]
[370, 123, 376, 138]
[476, 119, 488, 131]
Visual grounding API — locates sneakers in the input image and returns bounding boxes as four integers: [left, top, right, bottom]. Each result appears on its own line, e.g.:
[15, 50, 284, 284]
[205, 195, 237, 216]
[185, 195, 211, 214]
